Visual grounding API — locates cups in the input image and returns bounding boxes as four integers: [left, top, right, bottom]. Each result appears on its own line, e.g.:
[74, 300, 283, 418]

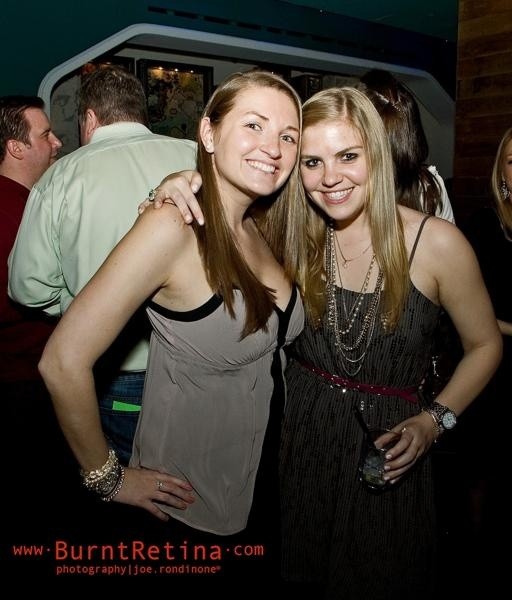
[358, 429, 402, 493]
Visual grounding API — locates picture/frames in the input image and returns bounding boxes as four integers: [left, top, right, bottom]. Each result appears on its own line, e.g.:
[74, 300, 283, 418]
[136, 57, 215, 139]
